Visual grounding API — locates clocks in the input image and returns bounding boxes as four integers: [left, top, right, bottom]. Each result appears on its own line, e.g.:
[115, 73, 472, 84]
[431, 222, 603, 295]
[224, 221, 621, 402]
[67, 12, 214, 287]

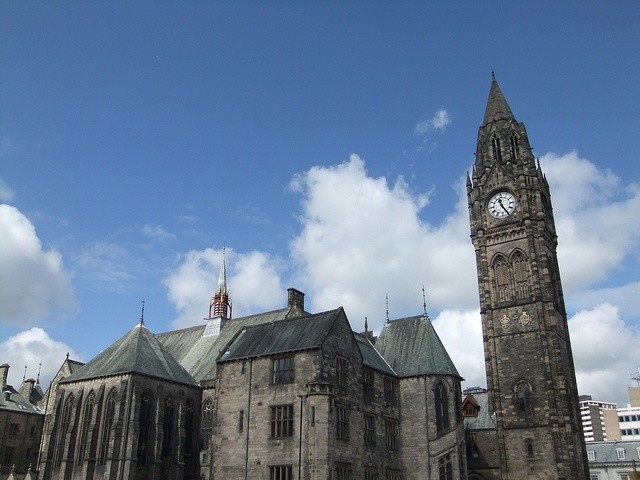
[485, 185, 518, 219]
